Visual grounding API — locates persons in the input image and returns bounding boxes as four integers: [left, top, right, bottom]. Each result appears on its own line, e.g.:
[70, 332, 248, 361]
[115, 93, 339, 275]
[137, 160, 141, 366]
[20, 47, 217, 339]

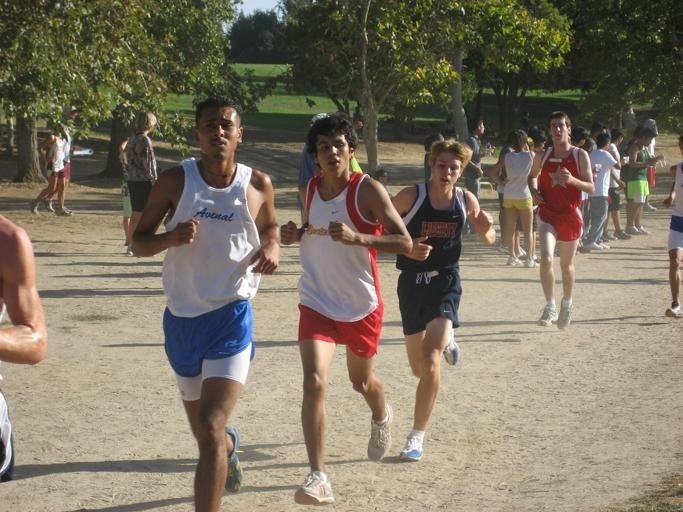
[119, 112, 158, 256]
[0, 215, 48, 484]
[33, 108, 78, 216]
[664, 134, 683, 318]
[494, 111, 664, 268]
[297, 113, 390, 225]
[527, 112, 595, 332]
[424, 134, 444, 183]
[463, 118, 488, 234]
[132, 95, 280, 512]
[382, 137, 496, 464]
[283, 115, 414, 508]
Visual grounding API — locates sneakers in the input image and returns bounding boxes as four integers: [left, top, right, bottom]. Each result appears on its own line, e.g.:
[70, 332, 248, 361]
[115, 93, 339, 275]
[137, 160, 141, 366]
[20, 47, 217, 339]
[538, 303, 558, 328]
[498, 243, 541, 269]
[223, 425, 244, 496]
[367, 403, 392, 463]
[582, 223, 650, 251]
[644, 201, 658, 214]
[557, 296, 574, 331]
[30, 197, 74, 216]
[664, 300, 681, 316]
[443, 329, 460, 368]
[294, 470, 335, 506]
[398, 429, 425, 463]
